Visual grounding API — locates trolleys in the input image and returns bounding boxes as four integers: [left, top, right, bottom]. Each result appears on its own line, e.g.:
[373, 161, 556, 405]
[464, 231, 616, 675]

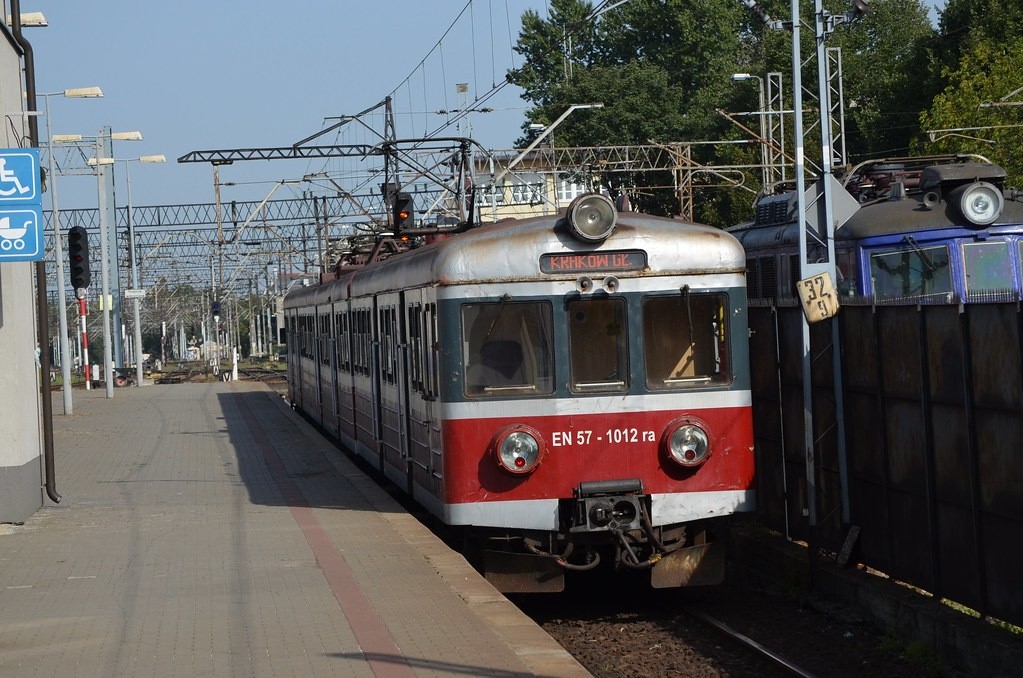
[112, 366, 138, 389]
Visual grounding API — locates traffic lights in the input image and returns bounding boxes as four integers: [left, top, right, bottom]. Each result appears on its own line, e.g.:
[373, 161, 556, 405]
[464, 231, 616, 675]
[211, 301, 220, 319]
[68, 226, 90, 290]
[392, 193, 417, 250]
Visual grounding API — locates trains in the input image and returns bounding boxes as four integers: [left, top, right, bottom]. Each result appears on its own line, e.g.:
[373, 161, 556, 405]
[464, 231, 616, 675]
[730, 151, 1023, 333]
[275, 194, 763, 611]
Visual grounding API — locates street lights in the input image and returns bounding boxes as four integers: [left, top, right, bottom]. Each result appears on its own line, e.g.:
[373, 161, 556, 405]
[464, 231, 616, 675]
[730, 72, 771, 196]
[85, 153, 168, 389]
[53, 129, 143, 399]
[33, 84, 106, 412]
